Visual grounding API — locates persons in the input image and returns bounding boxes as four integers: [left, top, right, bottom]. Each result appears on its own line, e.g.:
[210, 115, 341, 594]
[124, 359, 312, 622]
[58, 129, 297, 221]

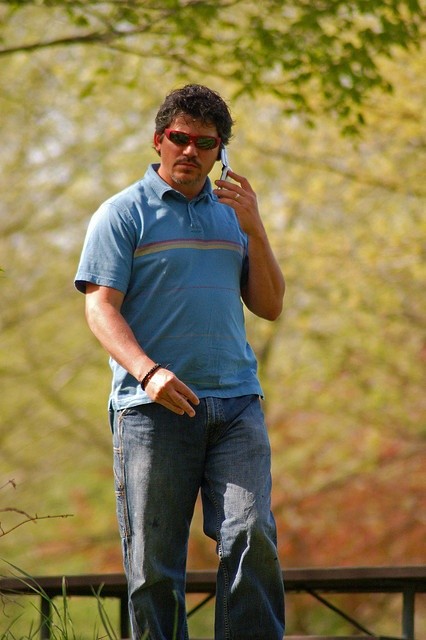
[74, 84, 286, 638]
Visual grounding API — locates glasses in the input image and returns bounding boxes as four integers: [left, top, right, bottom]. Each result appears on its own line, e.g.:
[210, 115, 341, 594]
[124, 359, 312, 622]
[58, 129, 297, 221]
[160, 125, 222, 151]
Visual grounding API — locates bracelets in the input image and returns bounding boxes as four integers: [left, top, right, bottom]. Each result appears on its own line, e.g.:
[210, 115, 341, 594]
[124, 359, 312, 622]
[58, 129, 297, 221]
[140, 363, 163, 391]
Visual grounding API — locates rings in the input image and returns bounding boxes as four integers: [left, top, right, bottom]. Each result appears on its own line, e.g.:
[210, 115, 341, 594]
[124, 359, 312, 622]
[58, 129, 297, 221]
[234, 193, 240, 201]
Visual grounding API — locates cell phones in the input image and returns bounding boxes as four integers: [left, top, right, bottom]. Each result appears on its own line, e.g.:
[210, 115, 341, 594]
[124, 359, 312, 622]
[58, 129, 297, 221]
[220, 146, 233, 188]
